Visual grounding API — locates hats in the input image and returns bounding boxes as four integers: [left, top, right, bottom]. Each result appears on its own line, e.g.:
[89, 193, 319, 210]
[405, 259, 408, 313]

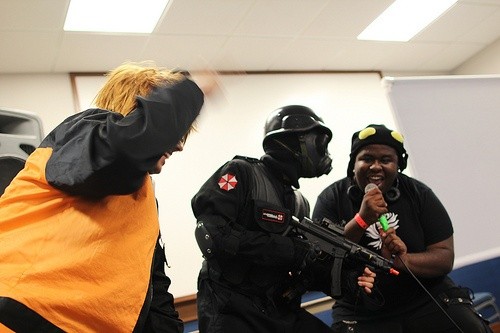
[347, 125, 408, 178]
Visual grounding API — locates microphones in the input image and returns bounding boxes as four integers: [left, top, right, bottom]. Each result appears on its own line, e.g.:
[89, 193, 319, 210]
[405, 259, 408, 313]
[364, 183, 399, 256]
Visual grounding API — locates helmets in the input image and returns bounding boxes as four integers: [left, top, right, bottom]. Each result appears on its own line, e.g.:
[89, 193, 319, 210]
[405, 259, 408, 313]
[263, 105, 332, 152]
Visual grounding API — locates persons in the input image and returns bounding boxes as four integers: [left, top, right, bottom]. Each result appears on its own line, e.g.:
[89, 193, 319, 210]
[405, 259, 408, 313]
[312, 124, 493, 333]
[192, 105, 375, 333]
[0, 64, 217, 333]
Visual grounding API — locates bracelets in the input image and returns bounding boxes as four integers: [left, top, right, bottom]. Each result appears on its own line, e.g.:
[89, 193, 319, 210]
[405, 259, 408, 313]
[354, 213, 367, 230]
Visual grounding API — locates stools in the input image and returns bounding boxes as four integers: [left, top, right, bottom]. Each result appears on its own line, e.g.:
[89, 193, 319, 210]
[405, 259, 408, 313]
[464, 292, 500, 323]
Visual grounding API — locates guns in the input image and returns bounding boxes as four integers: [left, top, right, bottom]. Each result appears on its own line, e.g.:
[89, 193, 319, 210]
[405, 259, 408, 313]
[281, 215, 399, 297]
